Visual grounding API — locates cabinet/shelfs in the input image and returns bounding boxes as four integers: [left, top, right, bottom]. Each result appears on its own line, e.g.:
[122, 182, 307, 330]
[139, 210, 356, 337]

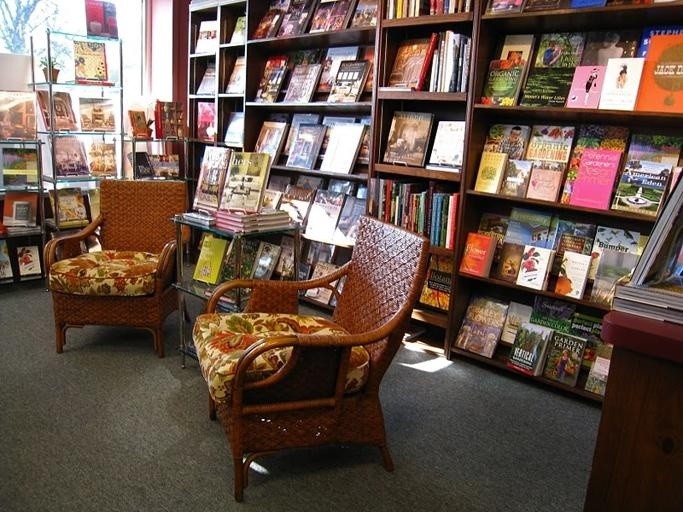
[0, 27, 188, 291]
[583, 311, 683, 511]
[186, 0, 681, 404]
[170, 214, 307, 370]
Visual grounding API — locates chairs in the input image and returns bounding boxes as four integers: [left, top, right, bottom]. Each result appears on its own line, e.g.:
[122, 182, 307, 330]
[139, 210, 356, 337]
[192, 215, 430, 503]
[43, 180, 191, 359]
